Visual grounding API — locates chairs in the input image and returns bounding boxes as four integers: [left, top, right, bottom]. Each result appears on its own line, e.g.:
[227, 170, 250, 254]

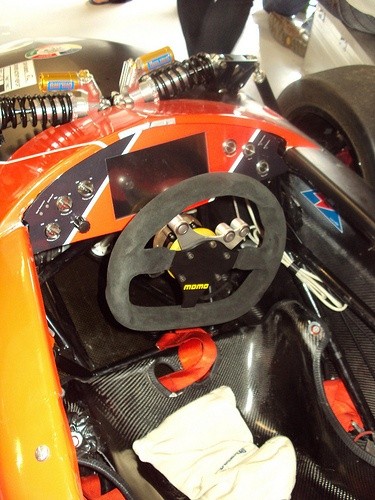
[84, 296, 375, 500]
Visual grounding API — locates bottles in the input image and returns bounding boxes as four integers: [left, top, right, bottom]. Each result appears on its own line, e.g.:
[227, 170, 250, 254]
[112, 50, 226, 104]
[0, 89, 111, 128]
